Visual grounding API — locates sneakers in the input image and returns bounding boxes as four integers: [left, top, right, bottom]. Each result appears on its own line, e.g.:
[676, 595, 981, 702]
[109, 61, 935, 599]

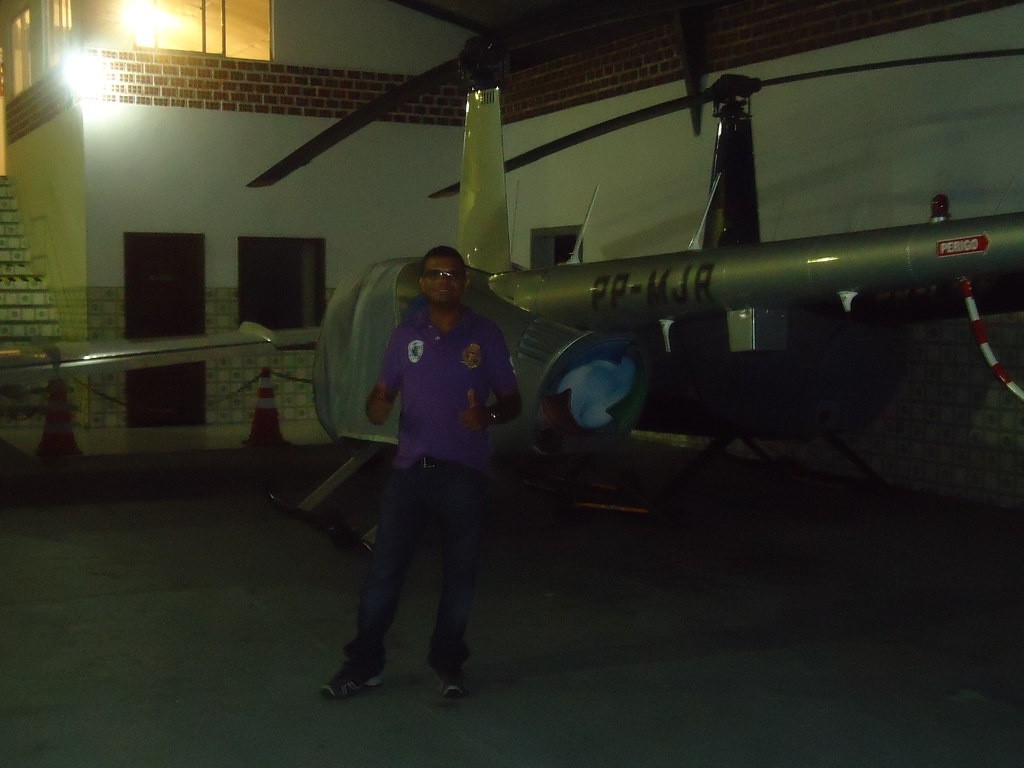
[321, 670, 381, 698]
[435, 663, 468, 697]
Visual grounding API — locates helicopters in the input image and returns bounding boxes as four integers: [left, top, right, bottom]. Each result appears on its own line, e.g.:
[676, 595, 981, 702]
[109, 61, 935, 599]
[0, 46, 1024, 559]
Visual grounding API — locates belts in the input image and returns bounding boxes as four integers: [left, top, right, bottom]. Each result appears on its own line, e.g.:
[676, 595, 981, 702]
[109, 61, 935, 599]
[412, 457, 442, 472]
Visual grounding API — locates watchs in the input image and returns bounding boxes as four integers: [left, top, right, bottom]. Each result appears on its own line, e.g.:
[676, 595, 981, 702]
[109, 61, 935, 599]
[487, 407, 500, 424]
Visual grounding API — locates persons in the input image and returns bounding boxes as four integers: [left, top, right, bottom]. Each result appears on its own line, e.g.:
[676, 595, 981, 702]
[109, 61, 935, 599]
[319, 246, 522, 701]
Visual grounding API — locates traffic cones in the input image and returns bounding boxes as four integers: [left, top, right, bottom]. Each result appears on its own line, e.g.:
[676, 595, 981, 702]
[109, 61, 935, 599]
[240, 366, 292, 448]
[32, 377, 85, 460]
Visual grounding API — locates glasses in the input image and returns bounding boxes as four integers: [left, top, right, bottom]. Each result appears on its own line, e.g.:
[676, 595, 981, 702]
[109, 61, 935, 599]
[425, 270, 465, 281]
